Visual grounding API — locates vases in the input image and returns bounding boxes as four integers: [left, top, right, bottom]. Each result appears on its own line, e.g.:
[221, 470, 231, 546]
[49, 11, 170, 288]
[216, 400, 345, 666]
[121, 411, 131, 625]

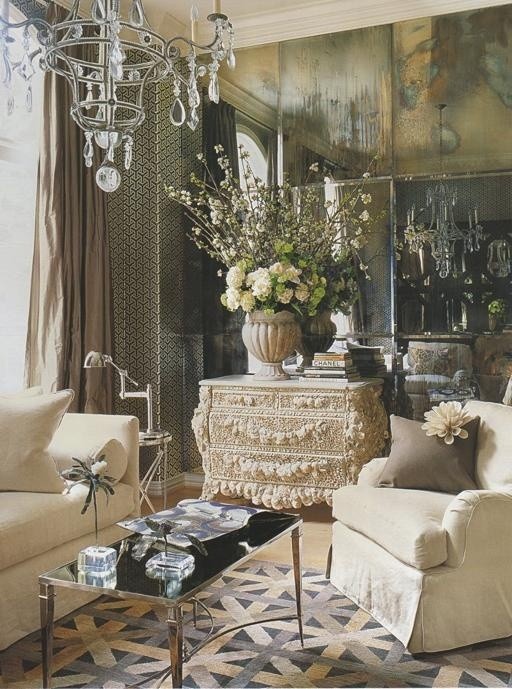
[297, 316, 339, 375]
[76, 546, 118, 569]
[241, 310, 304, 382]
[475, 374, 509, 404]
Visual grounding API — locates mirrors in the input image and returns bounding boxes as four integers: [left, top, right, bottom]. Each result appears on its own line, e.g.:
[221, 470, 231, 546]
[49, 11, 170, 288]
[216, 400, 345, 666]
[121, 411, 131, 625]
[231, 171, 512, 368]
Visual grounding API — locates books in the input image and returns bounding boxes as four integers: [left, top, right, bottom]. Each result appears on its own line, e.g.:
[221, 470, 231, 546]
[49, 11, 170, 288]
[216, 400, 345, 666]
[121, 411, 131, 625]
[298, 350, 361, 382]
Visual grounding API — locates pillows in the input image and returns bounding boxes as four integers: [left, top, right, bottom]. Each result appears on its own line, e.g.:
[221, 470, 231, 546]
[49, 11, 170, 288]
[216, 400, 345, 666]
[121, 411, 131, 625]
[379, 412, 481, 494]
[1, 388, 76, 495]
[408, 346, 452, 378]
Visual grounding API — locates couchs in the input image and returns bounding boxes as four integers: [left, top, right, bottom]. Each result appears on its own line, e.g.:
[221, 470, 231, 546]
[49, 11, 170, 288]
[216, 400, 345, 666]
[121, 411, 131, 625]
[501, 377, 512, 406]
[1, 384, 141, 652]
[326, 400, 511, 655]
[472, 333, 511, 363]
[405, 340, 473, 418]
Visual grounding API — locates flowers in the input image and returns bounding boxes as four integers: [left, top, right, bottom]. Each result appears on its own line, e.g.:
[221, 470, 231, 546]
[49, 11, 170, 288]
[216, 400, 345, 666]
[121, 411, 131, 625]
[321, 206, 381, 317]
[160, 131, 381, 319]
[477, 340, 511, 378]
[61, 453, 118, 550]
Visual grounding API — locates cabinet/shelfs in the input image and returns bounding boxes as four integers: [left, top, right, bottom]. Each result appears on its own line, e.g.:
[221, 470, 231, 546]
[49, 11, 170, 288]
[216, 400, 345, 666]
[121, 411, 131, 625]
[190, 372, 390, 509]
[362, 369, 407, 417]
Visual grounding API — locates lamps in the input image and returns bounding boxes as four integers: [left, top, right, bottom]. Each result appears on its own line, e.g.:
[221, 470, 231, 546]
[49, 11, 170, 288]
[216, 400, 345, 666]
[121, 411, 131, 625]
[395, 103, 486, 279]
[1, 0, 238, 194]
[81, 350, 170, 438]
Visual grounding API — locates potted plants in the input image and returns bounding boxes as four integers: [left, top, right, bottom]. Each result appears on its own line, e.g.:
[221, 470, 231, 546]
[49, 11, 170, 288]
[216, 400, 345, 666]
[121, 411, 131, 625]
[485, 298, 506, 332]
[117, 519, 210, 570]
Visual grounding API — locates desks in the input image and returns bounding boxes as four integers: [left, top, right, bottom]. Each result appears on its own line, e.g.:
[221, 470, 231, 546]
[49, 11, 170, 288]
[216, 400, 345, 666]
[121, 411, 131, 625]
[140, 427, 173, 513]
